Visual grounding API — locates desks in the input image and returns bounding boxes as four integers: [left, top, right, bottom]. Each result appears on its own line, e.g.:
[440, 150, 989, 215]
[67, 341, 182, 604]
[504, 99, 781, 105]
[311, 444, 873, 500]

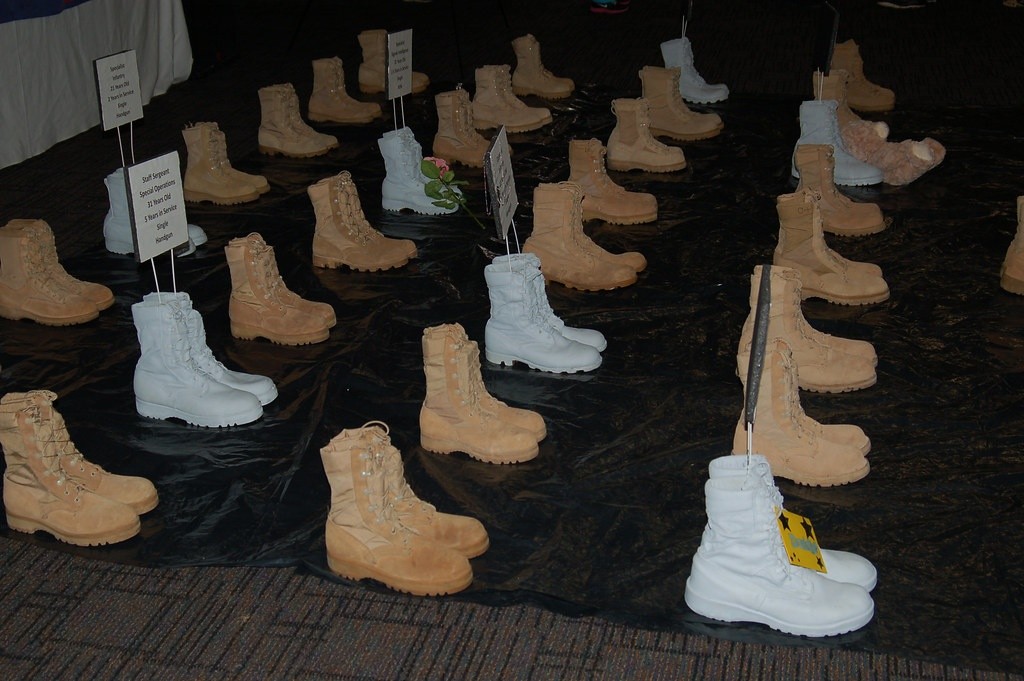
[0, 0, 195, 172]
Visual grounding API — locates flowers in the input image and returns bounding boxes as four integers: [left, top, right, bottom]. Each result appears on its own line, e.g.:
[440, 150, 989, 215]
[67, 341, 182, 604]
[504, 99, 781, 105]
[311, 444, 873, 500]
[421, 156, 486, 231]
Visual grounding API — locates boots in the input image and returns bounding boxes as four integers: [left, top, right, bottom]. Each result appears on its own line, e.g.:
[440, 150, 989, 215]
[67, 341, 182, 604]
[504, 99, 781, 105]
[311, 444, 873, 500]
[842, 120, 945, 184]
[829, 41, 894, 110]
[638, 65, 724, 140]
[471, 64, 551, 131]
[791, 99, 884, 185]
[795, 143, 886, 236]
[419, 323, 548, 464]
[432, 85, 514, 167]
[814, 69, 889, 138]
[521, 182, 647, 291]
[307, 56, 383, 122]
[310, 170, 419, 272]
[318, 419, 490, 596]
[377, 125, 462, 216]
[568, 140, 660, 224]
[482, 252, 608, 374]
[1, 123, 333, 546]
[729, 338, 872, 486]
[686, 454, 876, 636]
[737, 262, 878, 392]
[256, 83, 339, 155]
[512, 33, 575, 99]
[772, 187, 890, 306]
[605, 97, 687, 173]
[1001, 196, 1024, 293]
[660, 16, 729, 103]
[356, 29, 430, 93]
